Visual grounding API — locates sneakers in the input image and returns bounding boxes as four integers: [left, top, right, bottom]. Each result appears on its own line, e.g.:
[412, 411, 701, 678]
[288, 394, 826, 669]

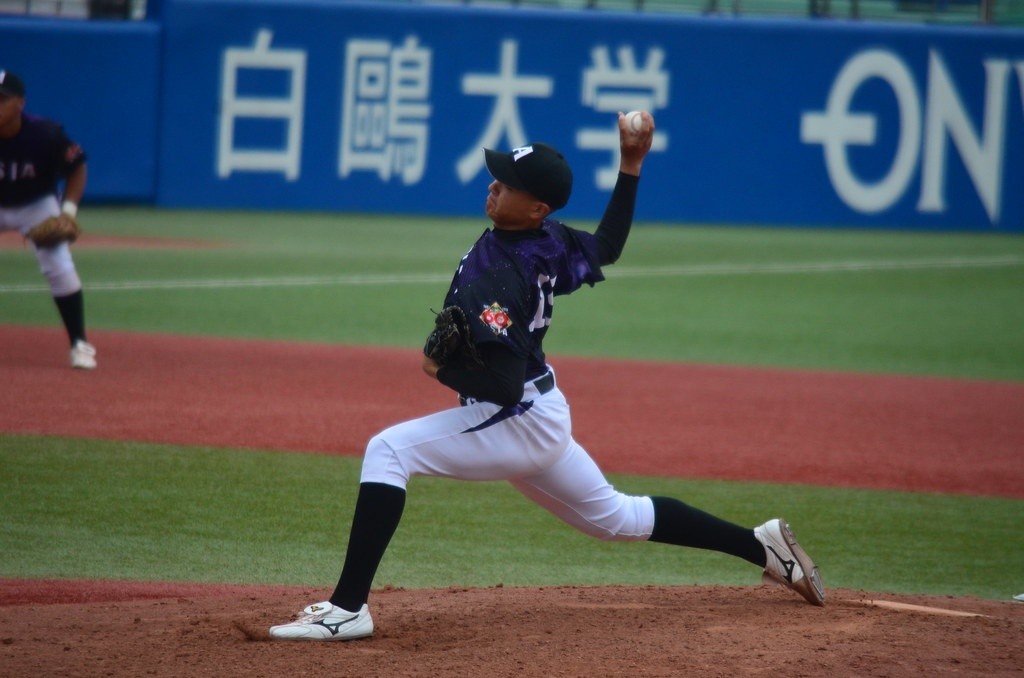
[70, 339, 96, 368]
[269, 601, 373, 641]
[754, 517, 825, 606]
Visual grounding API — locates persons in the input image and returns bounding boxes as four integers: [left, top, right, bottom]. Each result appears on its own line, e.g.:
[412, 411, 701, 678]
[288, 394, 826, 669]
[0, 66, 99, 372]
[269, 109, 827, 642]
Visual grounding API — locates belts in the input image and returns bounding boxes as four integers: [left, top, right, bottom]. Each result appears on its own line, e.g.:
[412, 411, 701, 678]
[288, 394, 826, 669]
[459, 372, 554, 407]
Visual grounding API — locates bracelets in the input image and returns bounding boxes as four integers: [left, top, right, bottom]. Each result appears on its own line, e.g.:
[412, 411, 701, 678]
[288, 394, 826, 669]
[62, 200, 77, 217]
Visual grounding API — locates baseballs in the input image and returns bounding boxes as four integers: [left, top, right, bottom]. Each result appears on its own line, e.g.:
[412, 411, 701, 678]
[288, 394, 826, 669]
[624, 110, 642, 136]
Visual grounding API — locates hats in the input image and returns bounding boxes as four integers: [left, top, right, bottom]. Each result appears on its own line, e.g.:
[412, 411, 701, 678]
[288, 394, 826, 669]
[0, 72, 24, 97]
[483, 143, 573, 205]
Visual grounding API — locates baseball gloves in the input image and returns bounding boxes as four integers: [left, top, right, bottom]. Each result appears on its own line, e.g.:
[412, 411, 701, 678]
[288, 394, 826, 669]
[423, 305, 471, 362]
[24, 215, 79, 249]
